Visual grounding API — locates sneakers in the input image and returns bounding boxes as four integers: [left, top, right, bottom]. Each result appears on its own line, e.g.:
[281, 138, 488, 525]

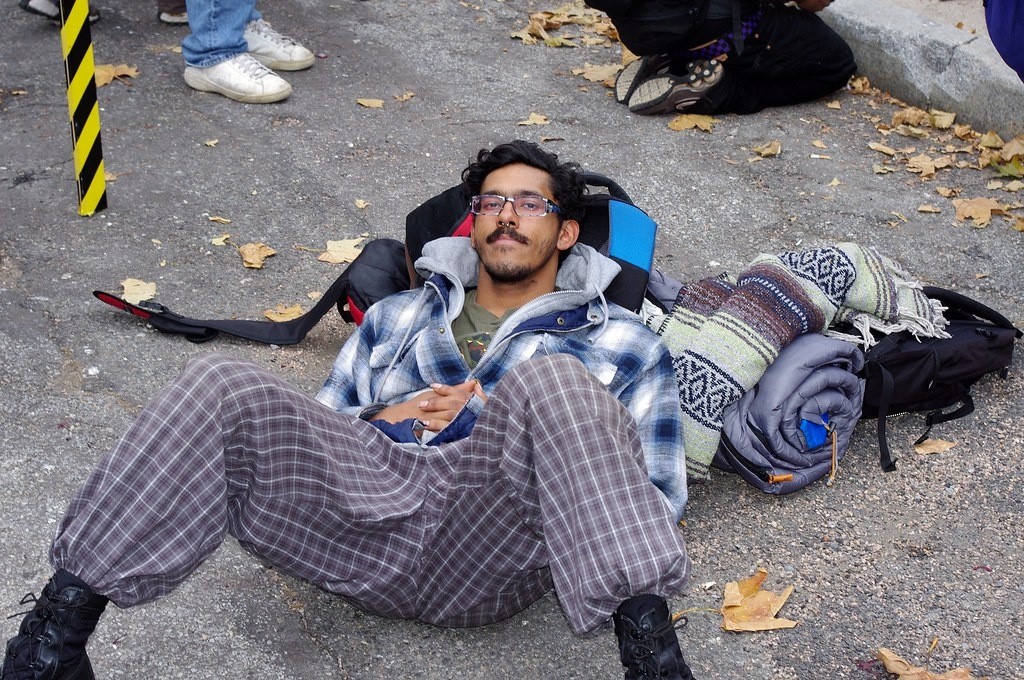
[161, 11, 189, 25]
[242, 19, 315, 70]
[19, 0, 99, 23]
[183, 51, 293, 103]
[613, 51, 676, 104]
[628, 59, 725, 116]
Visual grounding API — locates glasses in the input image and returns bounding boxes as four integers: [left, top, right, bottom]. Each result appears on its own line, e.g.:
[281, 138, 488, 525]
[470, 196, 564, 217]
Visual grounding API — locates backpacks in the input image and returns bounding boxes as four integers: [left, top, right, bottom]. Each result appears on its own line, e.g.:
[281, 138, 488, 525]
[825, 285, 1023, 474]
[92, 170, 658, 346]
[584, 1, 711, 54]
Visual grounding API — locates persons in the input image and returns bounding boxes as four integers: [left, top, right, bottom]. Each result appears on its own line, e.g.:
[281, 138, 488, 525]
[584, 0, 857, 115]
[0, 140, 695, 680]
[182, 0, 316, 102]
[17, 0, 188, 26]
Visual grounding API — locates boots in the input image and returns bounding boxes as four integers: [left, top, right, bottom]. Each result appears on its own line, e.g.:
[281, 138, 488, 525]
[611, 594, 696, 680]
[0, 568, 109, 680]
